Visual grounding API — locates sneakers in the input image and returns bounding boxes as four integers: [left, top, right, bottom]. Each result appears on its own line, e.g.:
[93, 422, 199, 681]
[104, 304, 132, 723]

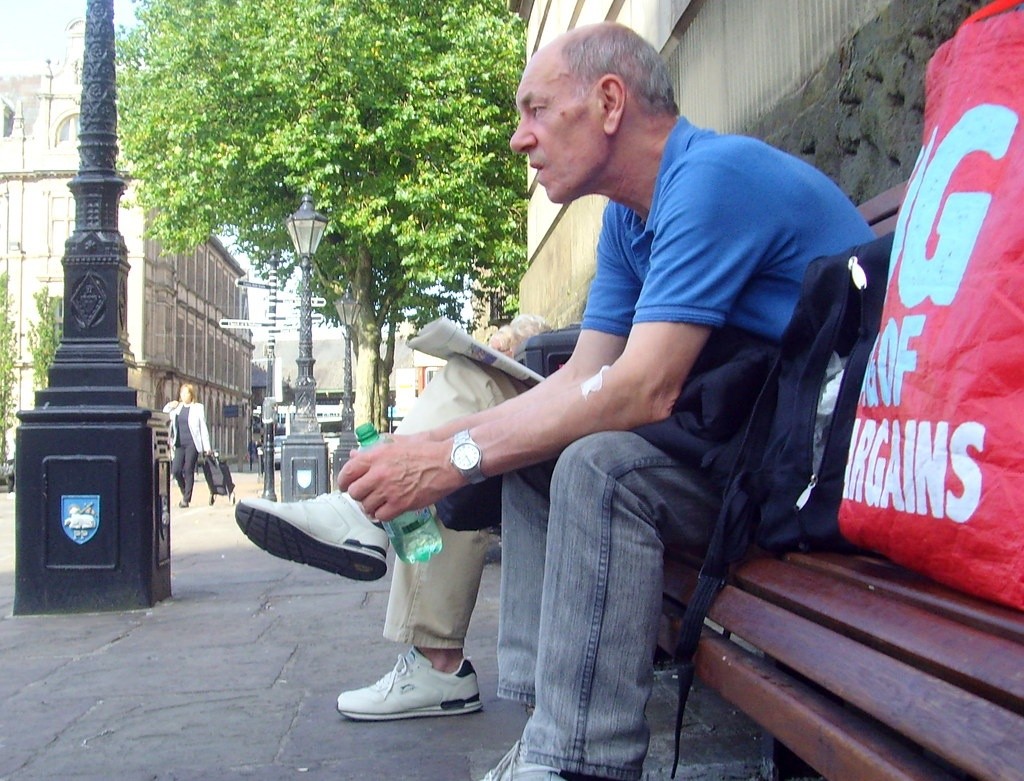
[337, 647, 483, 721]
[235, 489, 390, 581]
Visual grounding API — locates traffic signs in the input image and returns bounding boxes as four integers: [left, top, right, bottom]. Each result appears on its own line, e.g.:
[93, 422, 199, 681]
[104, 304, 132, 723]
[235, 278, 271, 291]
[276, 313, 323, 325]
[275, 296, 328, 308]
[218, 318, 272, 331]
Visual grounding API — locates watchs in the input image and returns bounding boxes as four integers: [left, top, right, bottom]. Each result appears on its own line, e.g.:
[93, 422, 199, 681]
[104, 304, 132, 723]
[449, 429, 487, 485]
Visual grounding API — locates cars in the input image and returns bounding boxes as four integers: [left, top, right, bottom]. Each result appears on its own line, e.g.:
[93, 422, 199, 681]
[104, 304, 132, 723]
[273, 435, 289, 470]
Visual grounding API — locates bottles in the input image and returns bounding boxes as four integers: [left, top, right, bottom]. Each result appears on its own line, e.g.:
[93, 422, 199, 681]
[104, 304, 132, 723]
[356, 423, 443, 564]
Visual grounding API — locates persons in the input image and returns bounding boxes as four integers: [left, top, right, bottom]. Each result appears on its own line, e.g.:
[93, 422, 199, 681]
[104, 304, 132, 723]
[5, 419, 19, 494]
[247, 440, 263, 463]
[163, 383, 212, 507]
[236, 311, 582, 722]
[337, 21, 875, 781]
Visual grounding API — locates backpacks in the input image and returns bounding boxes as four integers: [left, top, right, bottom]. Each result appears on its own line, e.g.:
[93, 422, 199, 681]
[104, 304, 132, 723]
[719, 233, 891, 554]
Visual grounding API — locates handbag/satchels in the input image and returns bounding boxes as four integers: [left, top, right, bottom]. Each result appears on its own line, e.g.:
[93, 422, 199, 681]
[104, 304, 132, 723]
[836, 0, 1024, 612]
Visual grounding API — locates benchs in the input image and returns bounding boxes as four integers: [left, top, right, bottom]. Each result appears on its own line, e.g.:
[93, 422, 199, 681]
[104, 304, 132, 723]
[655, 178, 1024, 781]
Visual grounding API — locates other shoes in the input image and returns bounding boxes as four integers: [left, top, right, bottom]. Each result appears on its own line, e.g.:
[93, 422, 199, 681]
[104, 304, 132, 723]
[480, 741, 567, 781]
[179, 500, 189, 508]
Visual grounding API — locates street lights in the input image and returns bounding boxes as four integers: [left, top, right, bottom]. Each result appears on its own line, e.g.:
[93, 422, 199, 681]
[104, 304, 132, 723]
[281, 195, 332, 504]
[332, 280, 364, 495]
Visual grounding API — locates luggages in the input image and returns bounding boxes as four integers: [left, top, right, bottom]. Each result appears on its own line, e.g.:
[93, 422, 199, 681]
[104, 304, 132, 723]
[203, 451, 236, 505]
